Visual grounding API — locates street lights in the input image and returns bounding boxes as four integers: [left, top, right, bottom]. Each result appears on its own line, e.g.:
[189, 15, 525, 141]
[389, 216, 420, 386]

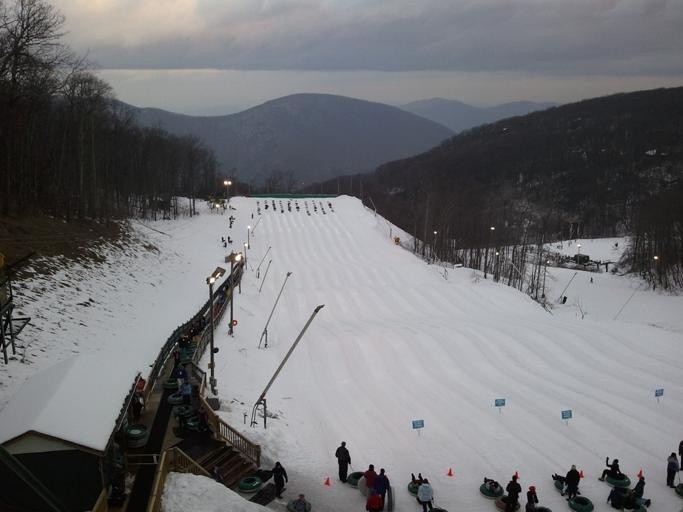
[223, 180, 232, 203]
[653, 255, 659, 289]
[225, 249, 242, 335]
[206, 266, 226, 396]
[577, 243, 582, 266]
[247, 225, 251, 249]
[243, 241, 247, 269]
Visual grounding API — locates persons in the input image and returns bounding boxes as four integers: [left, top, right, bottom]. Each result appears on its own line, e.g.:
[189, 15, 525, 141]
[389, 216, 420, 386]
[418, 478, 434, 511]
[268, 461, 288, 498]
[505, 475, 521, 512]
[606, 487, 621, 509]
[630, 476, 645, 498]
[374, 468, 389, 498]
[366, 488, 383, 511]
[565, 464, 580, 500]
[334, 441, 351, 482]
[551, 472, 566, 482]
[666, 452, 679, 487]
[363, 464, 377, 493]
[526, 485, 538, 511]
[598, 456, 619, 481]
[678, 440, 683, 470]
[483, 476, 497, 491]
[171, 328, 197, 402]
